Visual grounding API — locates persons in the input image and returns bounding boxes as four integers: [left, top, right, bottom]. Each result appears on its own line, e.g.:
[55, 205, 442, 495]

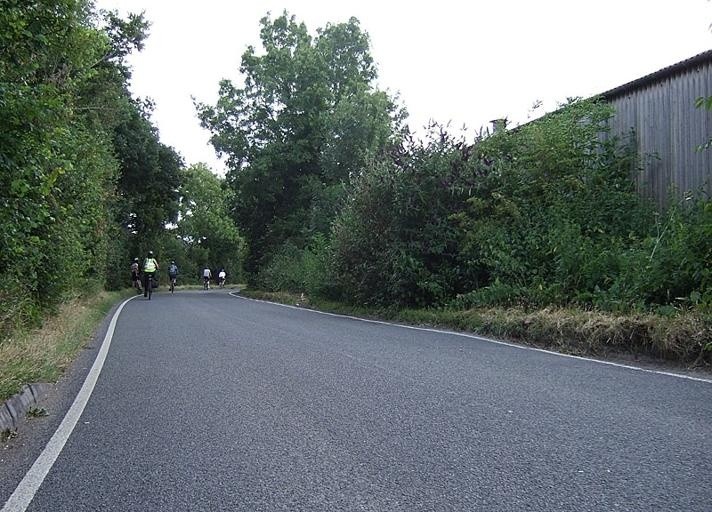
[140, 251, 159, 297]
[201, 266, 213, 291]
[130, 257, 139, 288]
[218, 267, 229, 289]
[167, 259, 180, 295]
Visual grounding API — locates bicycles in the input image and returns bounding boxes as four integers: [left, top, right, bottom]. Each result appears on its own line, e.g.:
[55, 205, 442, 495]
[218, 278, 226, 289]
[203, 276, 213, 291]
[133, 270, 142, 295]
[168, 272, 179, 294]
[142, 268, 161, 301]
